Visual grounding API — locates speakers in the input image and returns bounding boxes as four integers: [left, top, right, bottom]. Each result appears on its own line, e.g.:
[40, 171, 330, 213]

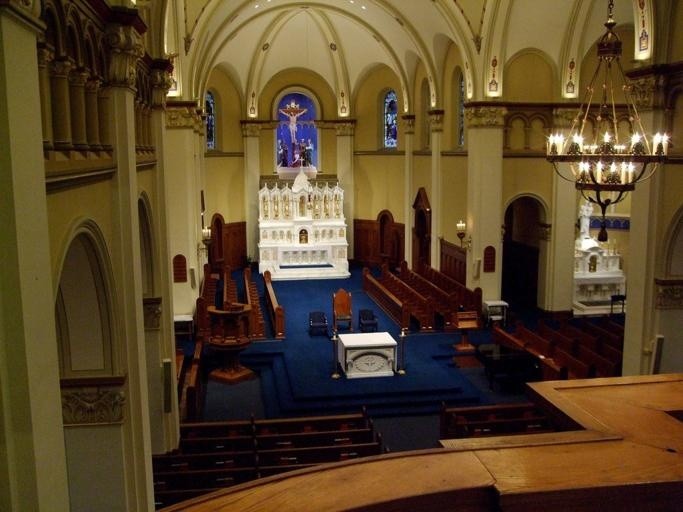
[189, 267, 196, 289]
[472, 258, 481, 278]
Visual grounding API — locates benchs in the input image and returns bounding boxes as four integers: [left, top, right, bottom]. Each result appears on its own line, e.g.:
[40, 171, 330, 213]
[153, 406, 389, 509]
[194, 262, 284, 340]
[439, 402, 583, 439]
[361, 261, 483, 333]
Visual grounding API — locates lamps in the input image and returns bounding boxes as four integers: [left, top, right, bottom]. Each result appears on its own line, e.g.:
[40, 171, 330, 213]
[456, 220, 471, 251]
[544, 0, 669, 242]
[197, 226, 212, 258]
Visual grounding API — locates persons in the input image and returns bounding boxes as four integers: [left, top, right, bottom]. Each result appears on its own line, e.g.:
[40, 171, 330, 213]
[577, 197, 593, 239]
[277, 138, 314, 167]
[278, 108, 308, 142]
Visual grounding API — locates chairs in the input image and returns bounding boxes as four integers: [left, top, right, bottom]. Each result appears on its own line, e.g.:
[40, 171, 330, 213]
[308, 311, 329, 336]
[332, 288, 353, 333]
[358, 308, 380, 332]
